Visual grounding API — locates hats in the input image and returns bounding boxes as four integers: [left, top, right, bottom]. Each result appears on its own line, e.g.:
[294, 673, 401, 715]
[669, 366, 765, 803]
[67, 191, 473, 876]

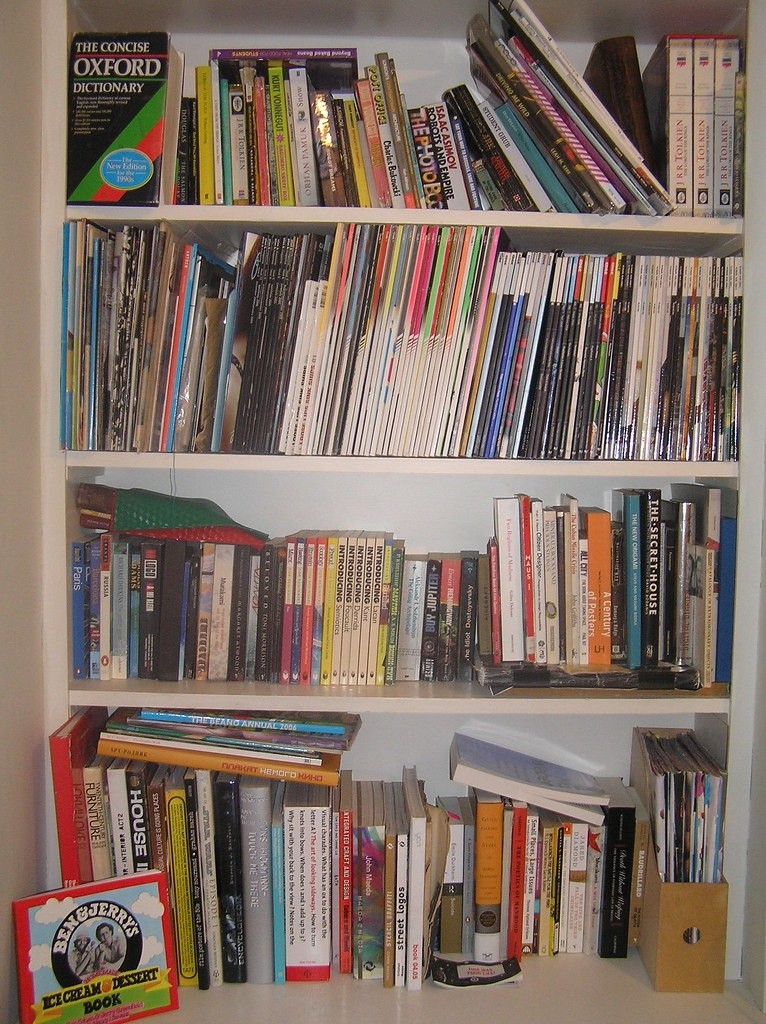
[96, 923, 114, 941]
[74, 931, 91, 944]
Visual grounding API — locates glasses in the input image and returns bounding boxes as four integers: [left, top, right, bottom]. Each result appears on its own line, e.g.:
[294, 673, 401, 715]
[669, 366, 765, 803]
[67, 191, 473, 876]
[76, 939, 89, 945]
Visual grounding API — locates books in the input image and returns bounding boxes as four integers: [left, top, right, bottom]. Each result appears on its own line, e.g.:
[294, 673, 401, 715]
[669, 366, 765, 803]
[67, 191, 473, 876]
[70, 466, 740, 700]
[12, 868, 179, 1024]
[59, 218, 745, 463]
[66, 0, 749, 219]
[48, 707, 730, 992]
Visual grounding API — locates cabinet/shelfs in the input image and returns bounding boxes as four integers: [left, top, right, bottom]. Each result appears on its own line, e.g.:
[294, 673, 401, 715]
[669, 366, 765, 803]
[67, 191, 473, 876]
[1, 0, 766, 979]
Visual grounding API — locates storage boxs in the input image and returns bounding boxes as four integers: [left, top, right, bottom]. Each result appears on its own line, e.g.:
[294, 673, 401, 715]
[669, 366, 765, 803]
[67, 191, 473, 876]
[637, 829, 728, 994]
